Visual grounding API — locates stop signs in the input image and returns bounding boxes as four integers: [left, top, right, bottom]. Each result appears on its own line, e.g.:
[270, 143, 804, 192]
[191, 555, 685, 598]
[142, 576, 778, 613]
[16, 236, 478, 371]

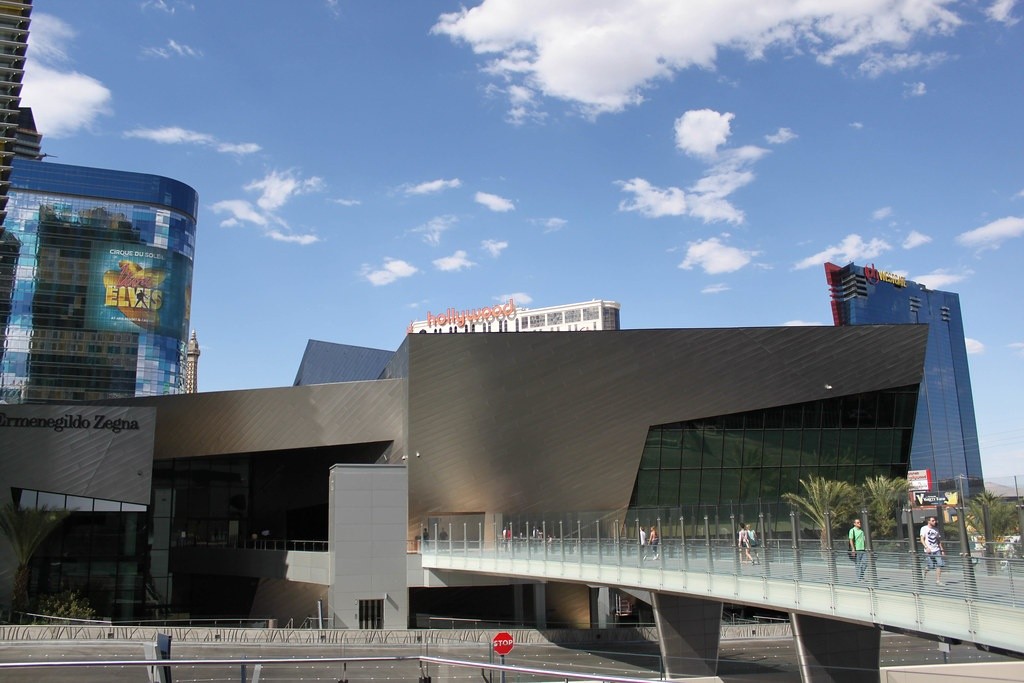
[493, 632, 514, 656]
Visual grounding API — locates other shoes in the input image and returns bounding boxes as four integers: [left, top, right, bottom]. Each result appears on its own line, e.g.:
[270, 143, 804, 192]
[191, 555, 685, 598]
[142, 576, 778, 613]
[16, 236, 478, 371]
[936, 581, 946, 587]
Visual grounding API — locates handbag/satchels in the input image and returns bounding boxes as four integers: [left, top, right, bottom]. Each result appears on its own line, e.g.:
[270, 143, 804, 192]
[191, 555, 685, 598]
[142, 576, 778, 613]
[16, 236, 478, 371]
[848, 549, 857, 562]
[749, 541, 757, 547]
[741, 542, 746, 547]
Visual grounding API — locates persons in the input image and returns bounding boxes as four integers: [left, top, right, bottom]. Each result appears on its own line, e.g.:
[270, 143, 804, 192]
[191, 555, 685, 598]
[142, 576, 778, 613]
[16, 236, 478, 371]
[738, 523, 755, 566]
[501, 526, 553, 553]
[743, 523, 761, 567]
[921, 513, 946, 588]
[646, 524, 661, 561]
[636, 525, 648, 562]
[847, 519, 871, 583]
